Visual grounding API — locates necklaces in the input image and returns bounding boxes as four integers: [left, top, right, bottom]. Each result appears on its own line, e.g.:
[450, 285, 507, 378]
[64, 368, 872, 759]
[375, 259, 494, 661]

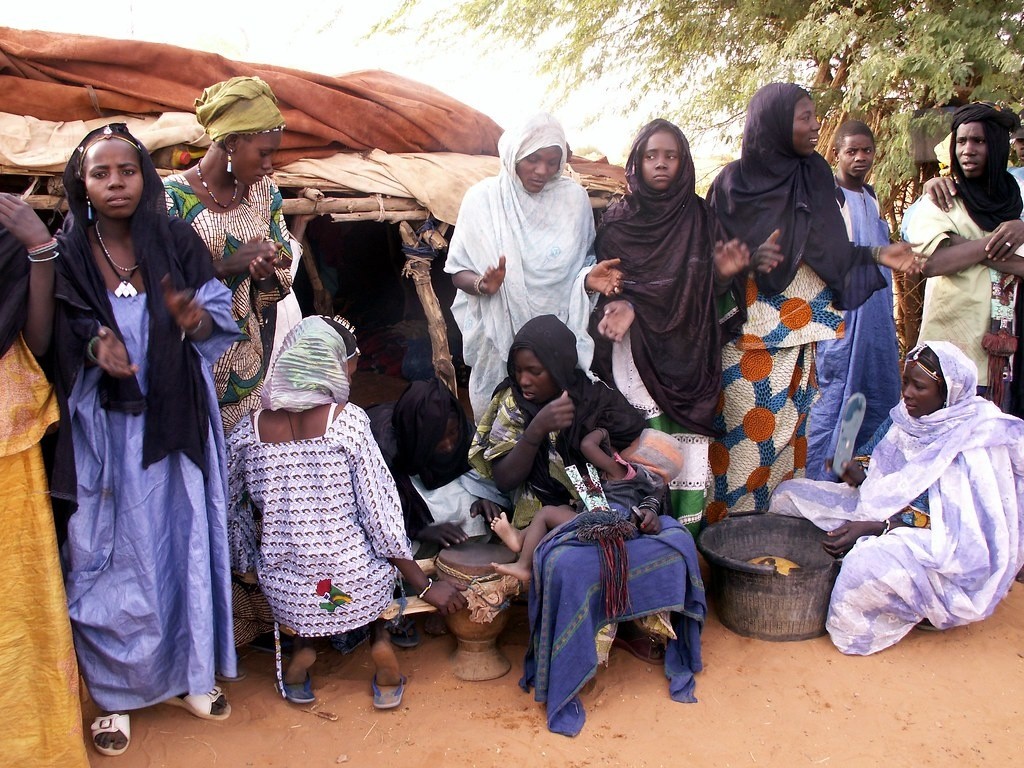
[95, 222, 140, 299]
[197, 157, 239, 208]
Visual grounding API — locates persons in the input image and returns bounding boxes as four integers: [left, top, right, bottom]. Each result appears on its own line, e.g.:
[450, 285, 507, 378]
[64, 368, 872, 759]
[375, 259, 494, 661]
[468, 313, 708, 738]
[923, 107, 1024, 419]
[767, 341, 1024, 657]
[489, 429, 684, 581]
[705, 83, 931, 528]
[40, 122, 251, 757]
[0, 191, 92, 768]
[588, 117, 750, 546]
[907, 102, 1024, 415]
[225, 313, 468, 708]
[806, 120, 901, 482]
[444, 111, 624, 428]
[390, 376, 525, 637]
[161, 74, 293, 645]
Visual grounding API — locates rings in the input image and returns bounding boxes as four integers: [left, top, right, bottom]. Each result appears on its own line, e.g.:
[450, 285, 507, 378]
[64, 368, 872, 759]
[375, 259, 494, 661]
[1006, 242, 1012, 247]
[261, 277, 266, 281]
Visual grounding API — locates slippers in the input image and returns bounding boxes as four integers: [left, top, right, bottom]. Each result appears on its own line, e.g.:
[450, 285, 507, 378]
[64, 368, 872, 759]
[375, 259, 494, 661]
[370, 670, 406, 709]
[277, 668, 315, 704]
[159, 684, 232, 721]
[90, 712, 131, 756]
[390, 616, 420, 648]
[612, 628, 667, 665]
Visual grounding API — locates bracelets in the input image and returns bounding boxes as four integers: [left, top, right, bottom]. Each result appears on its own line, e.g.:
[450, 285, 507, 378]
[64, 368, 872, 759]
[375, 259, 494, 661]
[474, 276, 488, 296]
[87, 336, 99, 363]
[881, 519, 891, 533]
[28, 238, 59, 262]
[181, 320, 202, 341]
[418, 577, 433, 598]
[596, 429, 608, 440]
[637, 496, 660, 515]
[876, 246, 882, 262]
[587, 290, 595, 296]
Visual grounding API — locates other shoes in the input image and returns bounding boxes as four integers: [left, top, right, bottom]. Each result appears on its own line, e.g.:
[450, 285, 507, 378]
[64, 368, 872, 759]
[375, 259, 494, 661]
[215, 669, 247, 682]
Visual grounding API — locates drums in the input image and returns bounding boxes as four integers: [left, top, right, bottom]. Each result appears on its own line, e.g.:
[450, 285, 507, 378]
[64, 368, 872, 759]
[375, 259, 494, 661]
[432, 542, 519, 682]
